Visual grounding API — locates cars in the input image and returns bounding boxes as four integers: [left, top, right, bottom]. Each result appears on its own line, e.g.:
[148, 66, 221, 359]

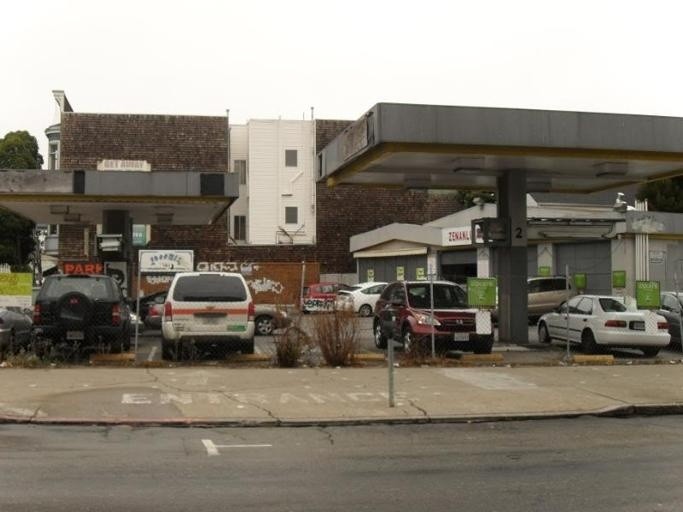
[1, 270, 294, 358]
